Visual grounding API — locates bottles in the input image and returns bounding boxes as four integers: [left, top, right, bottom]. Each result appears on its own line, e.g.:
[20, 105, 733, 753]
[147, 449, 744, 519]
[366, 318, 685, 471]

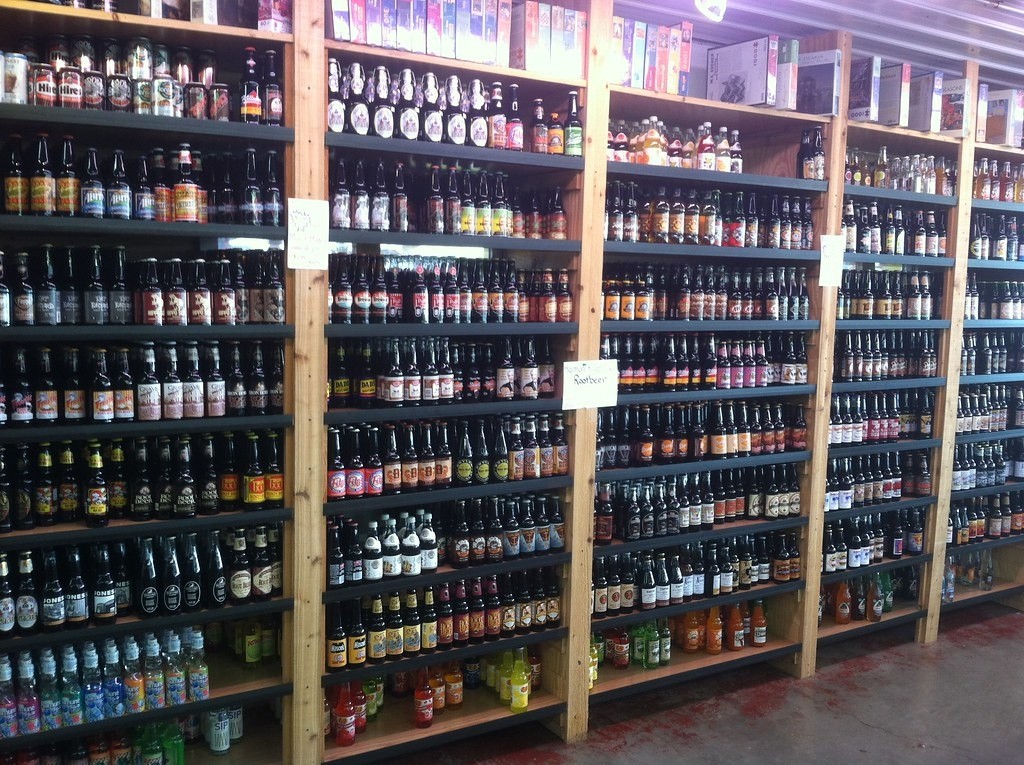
[1, 23, 1023, 763]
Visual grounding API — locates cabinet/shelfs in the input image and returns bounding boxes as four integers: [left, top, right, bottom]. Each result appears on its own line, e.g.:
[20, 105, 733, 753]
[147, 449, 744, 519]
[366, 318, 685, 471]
[1, 0, 1024, 765]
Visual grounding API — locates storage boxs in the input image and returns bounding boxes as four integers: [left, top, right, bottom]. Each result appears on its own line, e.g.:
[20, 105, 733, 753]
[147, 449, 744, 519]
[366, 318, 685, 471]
[324, 0, 1024, 148]
